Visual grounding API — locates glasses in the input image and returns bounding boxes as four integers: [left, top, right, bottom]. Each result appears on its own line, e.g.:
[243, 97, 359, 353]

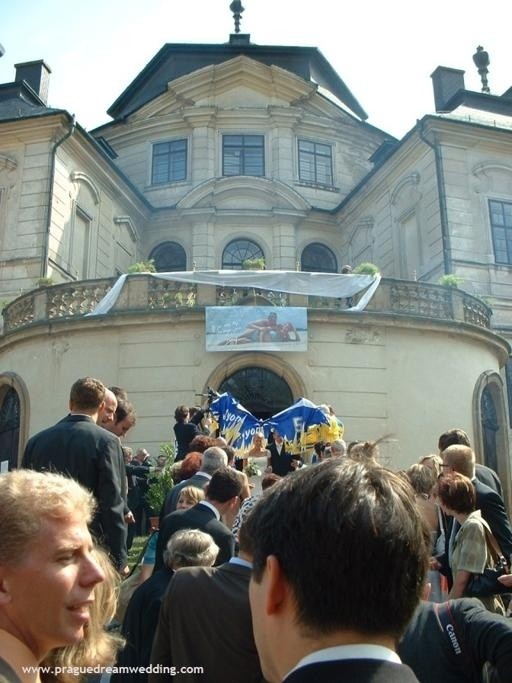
[439, 464, 449, 470]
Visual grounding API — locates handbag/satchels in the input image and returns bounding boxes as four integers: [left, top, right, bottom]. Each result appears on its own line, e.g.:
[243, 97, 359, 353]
[460, 517, 510, 596]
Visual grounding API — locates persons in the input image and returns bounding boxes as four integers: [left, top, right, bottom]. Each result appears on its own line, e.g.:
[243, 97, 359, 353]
[238, 456, 430, 683]
[188, 406, 213, 438]
[265, 428, 305, 478]
[137, 448, 157, 538]
[116, 482, 205, 637]
[243, 434, 269, 498]
[101, 398, 138, 442]
[232, 471, 283, 544]
[157, 444, 230, 538]
[396, 594, 512, 683]
[406, 459, 447, 603]
[119, 446, 164, 552]
[155, 455, 168, 472]
[115, 526, 219, 683]
[172, 404, 208, 463]
[0, 469, 108, 683]
[178, 449, 204, 482]
[222, 448, 260, 477]
[434, 472, 512, 613]
[217, 313, 300, 345]
[437, 429, 504, 506]
[20, 376, 134, 583]
[190, 433, 215, 454]
[39, 541, 124, 681]
[96, 386, 118, 434]
[419, 454, 445, 479]
[429, 445, 512, 610]
[106, 386, 126, 404]
[202, 468, 251, 530]
[155, 466, 246, 568]
[313, 440, 378, 462]
